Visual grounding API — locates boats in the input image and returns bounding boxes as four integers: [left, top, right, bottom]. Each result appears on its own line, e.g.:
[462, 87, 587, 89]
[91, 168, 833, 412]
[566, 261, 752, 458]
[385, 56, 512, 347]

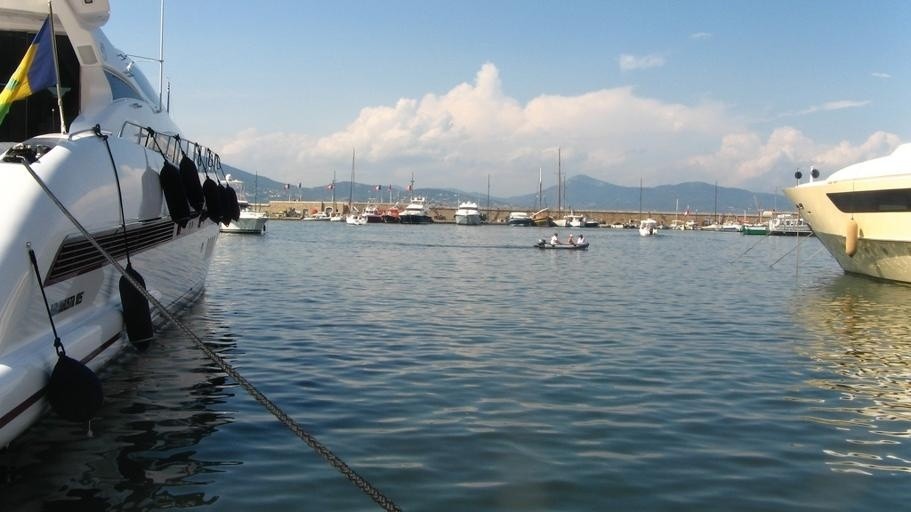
[639, 218, 657, 237]
[508, 146, 624, 229]
[671, 180, 813, 236]
[220, 174, 269, 234]
[454, 202, 481, 224]
[280, 149, 435, 225]
[0, 0, 223, 447]
[538, 239, 589, 249]
[783, 142, 911, 284]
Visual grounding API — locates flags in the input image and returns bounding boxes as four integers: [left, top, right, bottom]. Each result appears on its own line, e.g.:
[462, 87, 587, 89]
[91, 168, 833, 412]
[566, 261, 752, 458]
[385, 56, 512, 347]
[0, 14, 58, 125]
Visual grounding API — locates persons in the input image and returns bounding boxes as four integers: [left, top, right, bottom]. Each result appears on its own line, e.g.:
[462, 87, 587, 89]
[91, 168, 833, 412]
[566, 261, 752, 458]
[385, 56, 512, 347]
[578, 233, 587, 245]
[551, 233, 562, 245]
[568, 235, 575, 245]
